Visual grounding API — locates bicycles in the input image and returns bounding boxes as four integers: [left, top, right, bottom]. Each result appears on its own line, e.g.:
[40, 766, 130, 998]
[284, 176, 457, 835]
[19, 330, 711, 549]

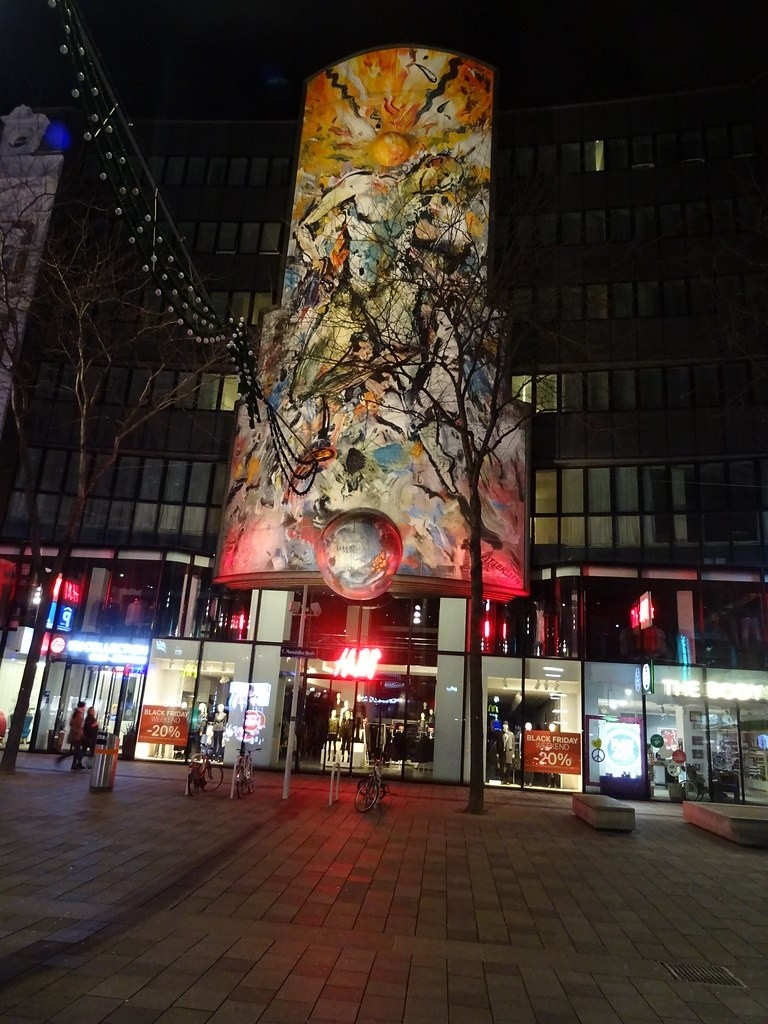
[234, 747, 262, 798]
[186, 742, 224, 797]
[354, 748, 390, 812]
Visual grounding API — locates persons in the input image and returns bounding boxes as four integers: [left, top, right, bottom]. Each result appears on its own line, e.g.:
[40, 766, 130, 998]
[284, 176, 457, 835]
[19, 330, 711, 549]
[174, 702, 185, 759]
[420, 702, 433, 726]
[55, 701, 85, 771]
[546, 723, 559, 788]
[497, 721, 514, 785]
[192, 703, 208, 760]
[519, 721, 533, 786]
[327, 693, 365, 761]
[210, 704, 227, 761]
[77, 708, 99, 770]
[154, 743, 165, 758]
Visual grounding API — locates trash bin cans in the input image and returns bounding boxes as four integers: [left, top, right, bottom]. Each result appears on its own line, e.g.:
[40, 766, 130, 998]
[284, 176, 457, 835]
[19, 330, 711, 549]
[89, 731, 120, 792]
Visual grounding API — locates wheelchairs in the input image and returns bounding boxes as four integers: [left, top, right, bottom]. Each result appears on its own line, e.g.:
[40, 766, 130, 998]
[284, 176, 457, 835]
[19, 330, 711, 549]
[674, 762, 728, 802]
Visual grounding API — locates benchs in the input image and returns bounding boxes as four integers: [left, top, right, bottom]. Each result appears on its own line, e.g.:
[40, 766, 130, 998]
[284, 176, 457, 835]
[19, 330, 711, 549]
[572, 794, 636, 831]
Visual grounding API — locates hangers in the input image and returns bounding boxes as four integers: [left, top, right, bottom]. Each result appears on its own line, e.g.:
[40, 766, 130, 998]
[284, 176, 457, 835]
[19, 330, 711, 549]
[132, 599, 144, 605]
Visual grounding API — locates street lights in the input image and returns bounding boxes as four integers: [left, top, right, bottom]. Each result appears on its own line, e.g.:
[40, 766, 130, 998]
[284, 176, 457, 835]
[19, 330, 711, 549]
[22, 561, 64, 753]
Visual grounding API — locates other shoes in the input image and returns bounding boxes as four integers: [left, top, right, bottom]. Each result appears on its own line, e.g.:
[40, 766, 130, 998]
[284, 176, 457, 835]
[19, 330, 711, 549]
[70, 762, 86, 771]
